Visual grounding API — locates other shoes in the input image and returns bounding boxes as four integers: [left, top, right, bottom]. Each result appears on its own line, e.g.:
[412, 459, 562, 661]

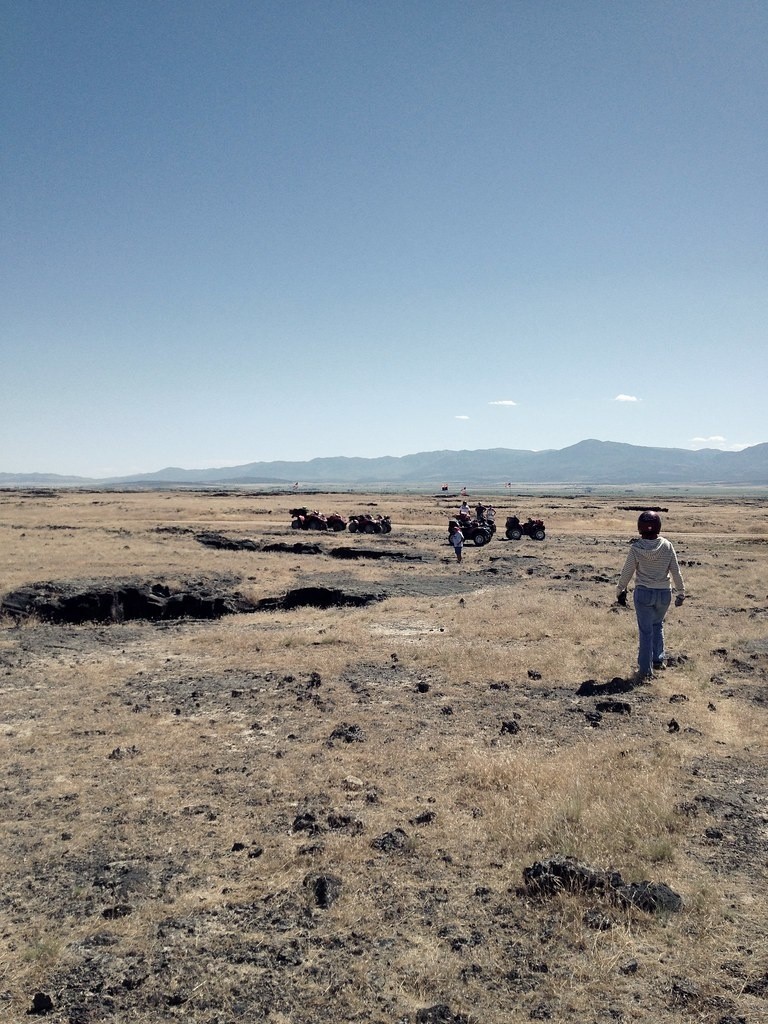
[653, 657, 667, 670]
[639, 672, 652, 684]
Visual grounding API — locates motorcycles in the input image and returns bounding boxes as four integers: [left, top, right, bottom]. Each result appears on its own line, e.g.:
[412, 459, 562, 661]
[448, 514, 496, 547]
[290, 506, 391, 534]
[505, 516, 546, 541]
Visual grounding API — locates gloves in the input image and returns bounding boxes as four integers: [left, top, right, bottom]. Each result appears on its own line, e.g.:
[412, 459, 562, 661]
[675, 594, 685, 607]
[618, 592, 626, 606]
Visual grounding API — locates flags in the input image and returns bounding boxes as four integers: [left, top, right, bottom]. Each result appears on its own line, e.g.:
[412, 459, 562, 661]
[505, 482, 511, 487]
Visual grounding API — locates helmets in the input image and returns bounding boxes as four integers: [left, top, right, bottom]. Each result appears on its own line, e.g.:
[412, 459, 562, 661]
[638, 511, 661, 534]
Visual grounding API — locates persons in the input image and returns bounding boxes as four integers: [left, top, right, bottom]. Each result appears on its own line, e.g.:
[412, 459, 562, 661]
[459, 501, 471, 516]
[615, 510, 685, 678]
[453, 527, 464, 563]
[487, 505, 496, 520]
[476, 502, 486, 519]
